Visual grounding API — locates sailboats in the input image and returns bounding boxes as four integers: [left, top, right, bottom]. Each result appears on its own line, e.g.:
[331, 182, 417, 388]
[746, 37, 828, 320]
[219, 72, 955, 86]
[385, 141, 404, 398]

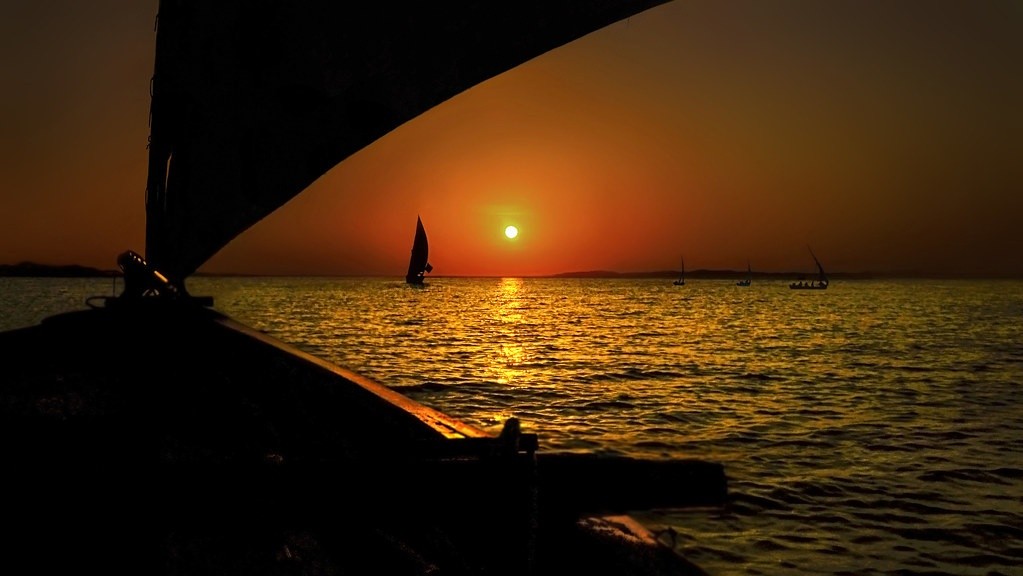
[673, 257, 685, 285]
[737, 262, 752, 287]
[404, 213, 433, 284]
[788, 245, 829, 290]
